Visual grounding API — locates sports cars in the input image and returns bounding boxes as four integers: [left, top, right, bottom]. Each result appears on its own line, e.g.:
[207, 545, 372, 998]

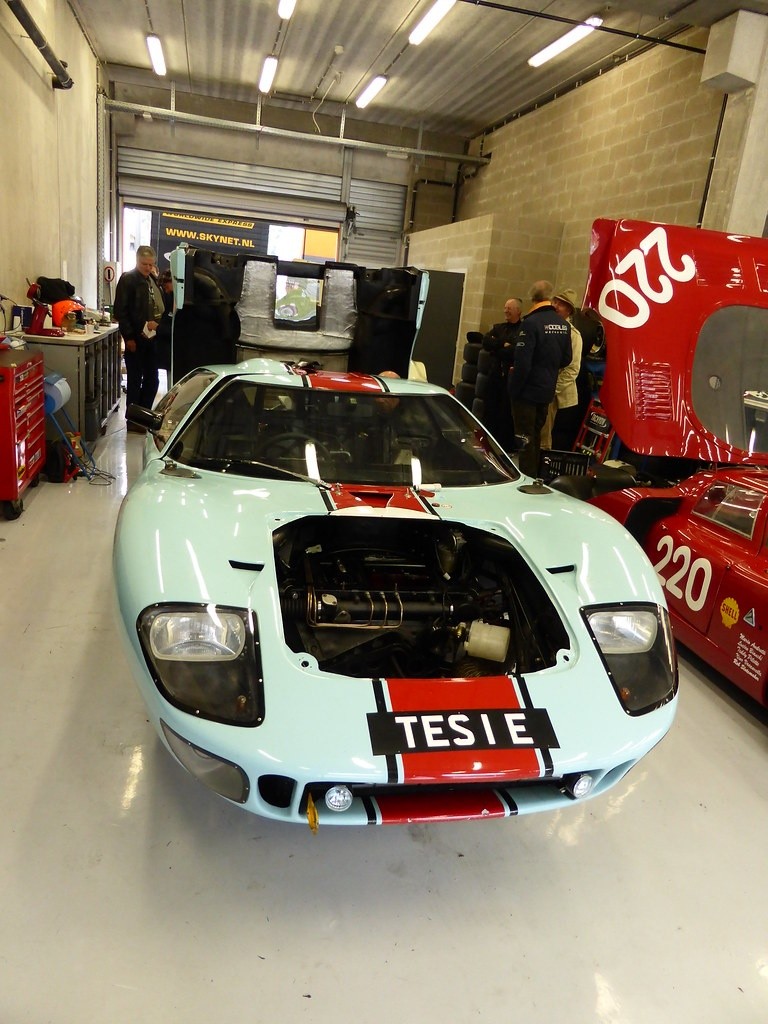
[546, 218, 767, 720]
[112, 358, 677, 827]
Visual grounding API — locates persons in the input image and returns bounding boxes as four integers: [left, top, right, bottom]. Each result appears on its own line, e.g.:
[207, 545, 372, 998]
[508, 282, 573, 431]
[541, 289, 582, 465]
[113, 245, 173, 433]
[552, 308, 607, 450]
[484, 298, 523, 459]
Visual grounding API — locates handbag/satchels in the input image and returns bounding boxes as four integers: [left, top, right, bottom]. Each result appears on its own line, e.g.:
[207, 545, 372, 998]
[36, 276, 75, 304]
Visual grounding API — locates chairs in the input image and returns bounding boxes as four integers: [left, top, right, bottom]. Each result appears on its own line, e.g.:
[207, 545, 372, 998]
[537, 449, 589, 483]
[378, 397, 446, 468]
[192, 390, 255, 466]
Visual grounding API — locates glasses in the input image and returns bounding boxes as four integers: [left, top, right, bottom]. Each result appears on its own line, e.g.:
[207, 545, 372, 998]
[160, 279, 170, 283]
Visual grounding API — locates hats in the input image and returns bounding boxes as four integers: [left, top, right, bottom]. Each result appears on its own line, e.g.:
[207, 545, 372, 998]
[555, 289, 577, 316]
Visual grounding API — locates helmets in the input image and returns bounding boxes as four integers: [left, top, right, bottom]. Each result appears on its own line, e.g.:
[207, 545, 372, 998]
[51, 300, 85, 327]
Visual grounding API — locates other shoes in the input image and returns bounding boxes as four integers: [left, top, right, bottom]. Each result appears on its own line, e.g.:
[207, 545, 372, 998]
[126, 420, 147, 432]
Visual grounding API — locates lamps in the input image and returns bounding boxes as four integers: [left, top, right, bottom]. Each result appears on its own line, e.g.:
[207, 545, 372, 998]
[277, 0, 298, 20]
[526, 14, 605, 67]
[146, 33, 167, 77]
[258, 55, 279, 94]
[355, 74, 388, 109]
[408, 0, 459, 45]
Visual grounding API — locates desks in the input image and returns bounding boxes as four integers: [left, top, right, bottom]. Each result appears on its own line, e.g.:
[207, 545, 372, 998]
[0, 319, 123, 443]
